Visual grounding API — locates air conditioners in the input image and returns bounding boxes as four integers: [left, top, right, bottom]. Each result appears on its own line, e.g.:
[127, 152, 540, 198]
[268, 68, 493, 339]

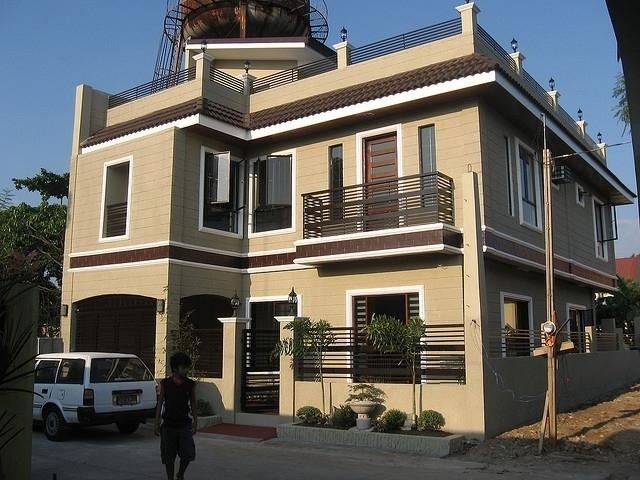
[552, 164, 571, 185]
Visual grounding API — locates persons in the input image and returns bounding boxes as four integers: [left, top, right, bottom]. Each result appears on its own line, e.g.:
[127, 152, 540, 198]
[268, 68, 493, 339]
[154, 352, 197, 480]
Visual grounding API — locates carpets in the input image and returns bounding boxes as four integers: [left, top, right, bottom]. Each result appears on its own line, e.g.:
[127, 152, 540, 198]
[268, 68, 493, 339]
[197, 423, 277, 440]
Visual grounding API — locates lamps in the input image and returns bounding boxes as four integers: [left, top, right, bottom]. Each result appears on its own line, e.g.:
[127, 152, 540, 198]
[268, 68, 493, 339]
[157, 299, 164, 312]
[61, 305, 67, 316]
[243, 60, 251, 73]
[549, 77, 556, 91]
[510, 37, 518, 52]
[340, 26, 347, 41]
[287, 285, 297, 315]
[577, 109, 584, 121]
[230, 288, 240, 317]
[201, 39, 208, 52]
[597, 132, 602, 144]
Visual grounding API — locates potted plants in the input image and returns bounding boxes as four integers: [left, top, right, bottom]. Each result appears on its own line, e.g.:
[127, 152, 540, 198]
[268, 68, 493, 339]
[346, 384, 386, 431]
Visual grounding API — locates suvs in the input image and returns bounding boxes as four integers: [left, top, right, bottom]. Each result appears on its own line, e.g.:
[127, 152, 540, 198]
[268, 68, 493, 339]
[33, 352, 159, 441]
